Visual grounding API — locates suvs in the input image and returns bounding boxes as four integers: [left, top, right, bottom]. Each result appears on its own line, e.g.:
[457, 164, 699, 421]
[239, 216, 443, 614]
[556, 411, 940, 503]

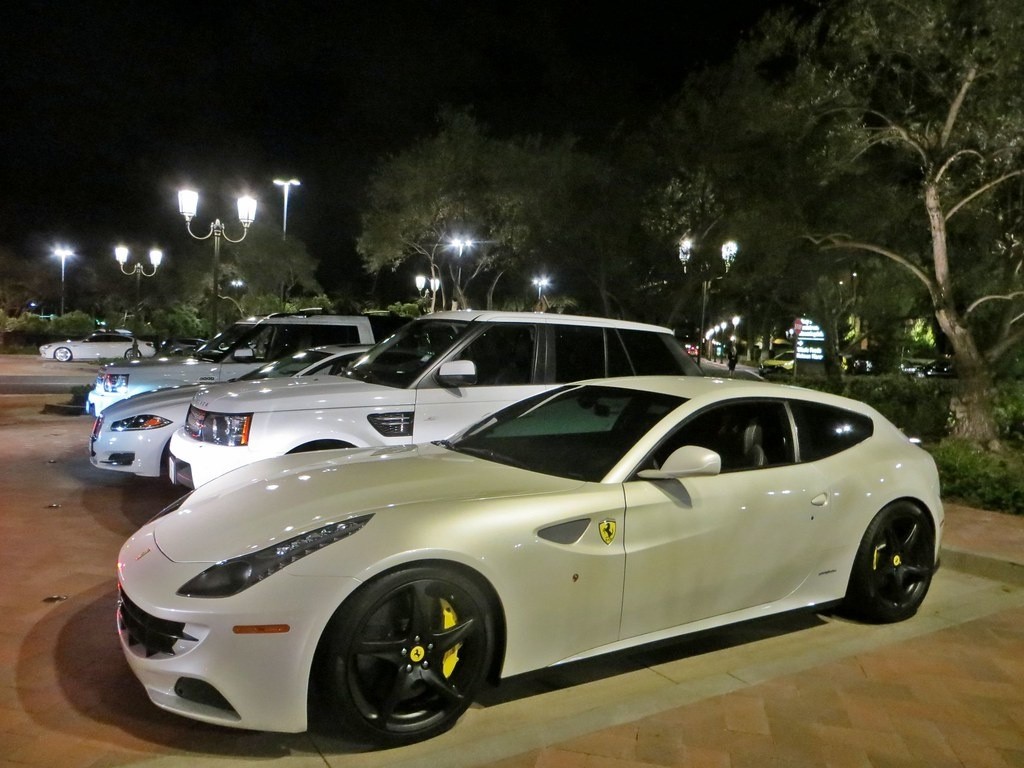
[167, 308, 712, 492]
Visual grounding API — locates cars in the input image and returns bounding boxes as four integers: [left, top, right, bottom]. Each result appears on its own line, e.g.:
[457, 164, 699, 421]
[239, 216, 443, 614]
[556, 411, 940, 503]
[847, 347, 873, 374]
[901, 348, 955, 381]
[83, 309, 472, 478]
[117, 376, 947, 746]
[39, 328, 157, 364]
[760, 350, 795, 377]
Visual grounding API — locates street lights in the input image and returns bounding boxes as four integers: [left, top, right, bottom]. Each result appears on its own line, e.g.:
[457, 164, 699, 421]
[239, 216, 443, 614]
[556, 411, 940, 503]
[54, 249, 72, 316]
[177, 189, 262, 348]
[679, 233, 738, 367]
[114, 244, 163, 325]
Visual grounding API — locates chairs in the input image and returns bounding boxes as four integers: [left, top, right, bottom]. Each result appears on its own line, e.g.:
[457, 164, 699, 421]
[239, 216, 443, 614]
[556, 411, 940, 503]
[683, 413, 762, 468]
[471, 351, 519, 386]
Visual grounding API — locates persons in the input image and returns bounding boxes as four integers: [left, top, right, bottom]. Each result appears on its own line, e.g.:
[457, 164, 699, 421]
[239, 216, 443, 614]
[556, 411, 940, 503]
[724, 339, 738, 377]
